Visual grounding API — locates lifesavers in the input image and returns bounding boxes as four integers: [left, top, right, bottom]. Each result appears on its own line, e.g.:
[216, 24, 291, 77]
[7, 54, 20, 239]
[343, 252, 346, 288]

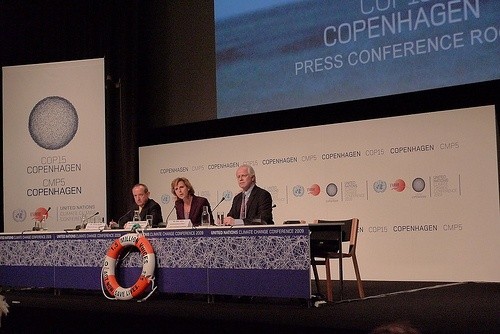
[102, 233, 155, 301]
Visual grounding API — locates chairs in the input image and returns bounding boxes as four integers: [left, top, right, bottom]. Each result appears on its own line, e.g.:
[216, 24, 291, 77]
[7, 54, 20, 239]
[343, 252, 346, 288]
[315, 218, 366, 301]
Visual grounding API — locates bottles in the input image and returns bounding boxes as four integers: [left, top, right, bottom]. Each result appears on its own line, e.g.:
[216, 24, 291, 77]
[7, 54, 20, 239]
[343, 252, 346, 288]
[133, 210, 141, 221]
[42, 215, 48, 231]
[81, 216, 87, 230]
[201, 206, 210, 227]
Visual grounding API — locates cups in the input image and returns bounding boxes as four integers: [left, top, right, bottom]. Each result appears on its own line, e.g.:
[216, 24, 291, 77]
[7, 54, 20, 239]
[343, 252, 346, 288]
[146, 215, 152, 228]
[217, 211, 224, 226]
[35, 221, 40, 231]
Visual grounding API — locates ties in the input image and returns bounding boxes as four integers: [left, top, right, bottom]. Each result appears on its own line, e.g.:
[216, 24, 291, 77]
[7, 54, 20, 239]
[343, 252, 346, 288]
[241, 193, 246, 219]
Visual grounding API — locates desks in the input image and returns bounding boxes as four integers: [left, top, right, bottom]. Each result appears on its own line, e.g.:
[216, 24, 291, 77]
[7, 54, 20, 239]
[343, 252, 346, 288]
[0, 222, 344, 308]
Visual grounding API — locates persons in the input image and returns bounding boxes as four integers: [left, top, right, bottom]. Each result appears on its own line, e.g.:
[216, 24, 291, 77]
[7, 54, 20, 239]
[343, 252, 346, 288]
[216, 165, 274, 225]
[371, 320, 422, 334]
[171, 178, 214, 226]
[109, 184, 163, 230]
